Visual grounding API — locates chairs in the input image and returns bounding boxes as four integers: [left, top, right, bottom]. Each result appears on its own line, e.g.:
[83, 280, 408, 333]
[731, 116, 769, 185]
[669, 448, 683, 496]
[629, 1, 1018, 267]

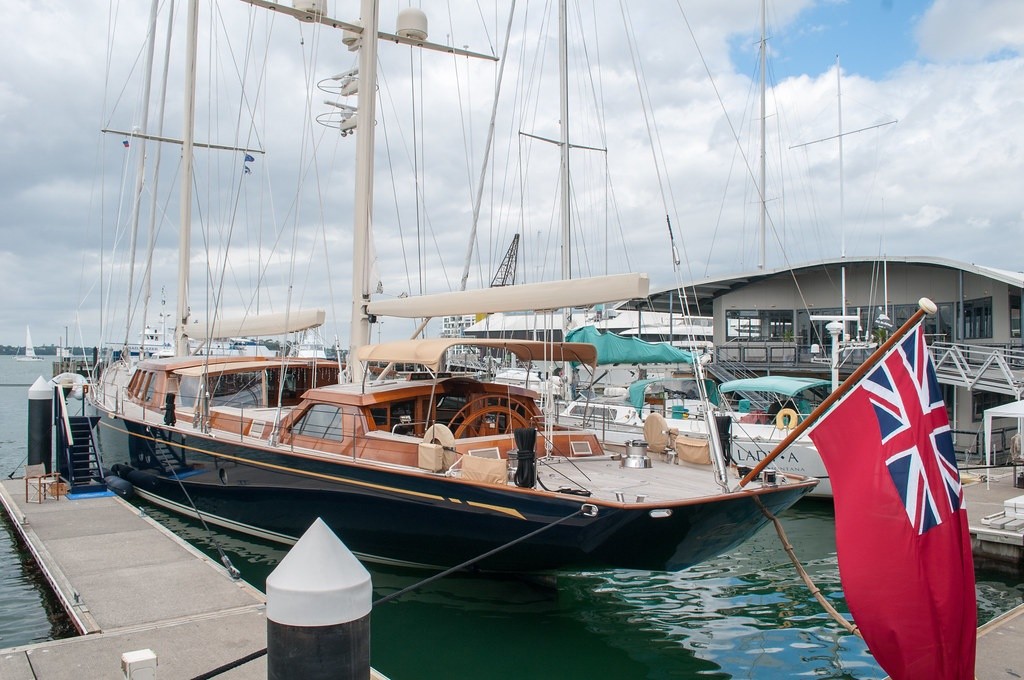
[23, 462, 61, 504]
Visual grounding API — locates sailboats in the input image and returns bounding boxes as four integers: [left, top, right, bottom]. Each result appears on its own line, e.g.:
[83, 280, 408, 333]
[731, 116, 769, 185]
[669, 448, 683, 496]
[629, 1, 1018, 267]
[458, 0, 833, 344]
[90, 0, 822, 578]
[13, 324, 44, 361]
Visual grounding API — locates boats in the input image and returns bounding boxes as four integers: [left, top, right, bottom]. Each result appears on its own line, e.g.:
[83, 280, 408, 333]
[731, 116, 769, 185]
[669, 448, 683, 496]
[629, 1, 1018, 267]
[547, 375, 834, 498]
[960, 399, 1024, 574]
[116, 286, 272, 358]
[53, 327, 108, 380]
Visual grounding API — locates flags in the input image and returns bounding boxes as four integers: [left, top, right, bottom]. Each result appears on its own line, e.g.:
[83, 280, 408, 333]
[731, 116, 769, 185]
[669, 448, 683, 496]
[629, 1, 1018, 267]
[807, 323, 976, 679]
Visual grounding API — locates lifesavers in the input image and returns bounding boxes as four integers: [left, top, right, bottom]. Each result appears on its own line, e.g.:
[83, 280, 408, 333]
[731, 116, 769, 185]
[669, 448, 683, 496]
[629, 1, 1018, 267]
[775, 407, 798, 430]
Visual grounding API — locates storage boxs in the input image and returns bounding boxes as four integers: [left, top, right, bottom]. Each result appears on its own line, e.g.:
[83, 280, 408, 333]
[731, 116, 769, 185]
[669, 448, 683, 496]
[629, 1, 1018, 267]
[48, 483, 68, 496]
